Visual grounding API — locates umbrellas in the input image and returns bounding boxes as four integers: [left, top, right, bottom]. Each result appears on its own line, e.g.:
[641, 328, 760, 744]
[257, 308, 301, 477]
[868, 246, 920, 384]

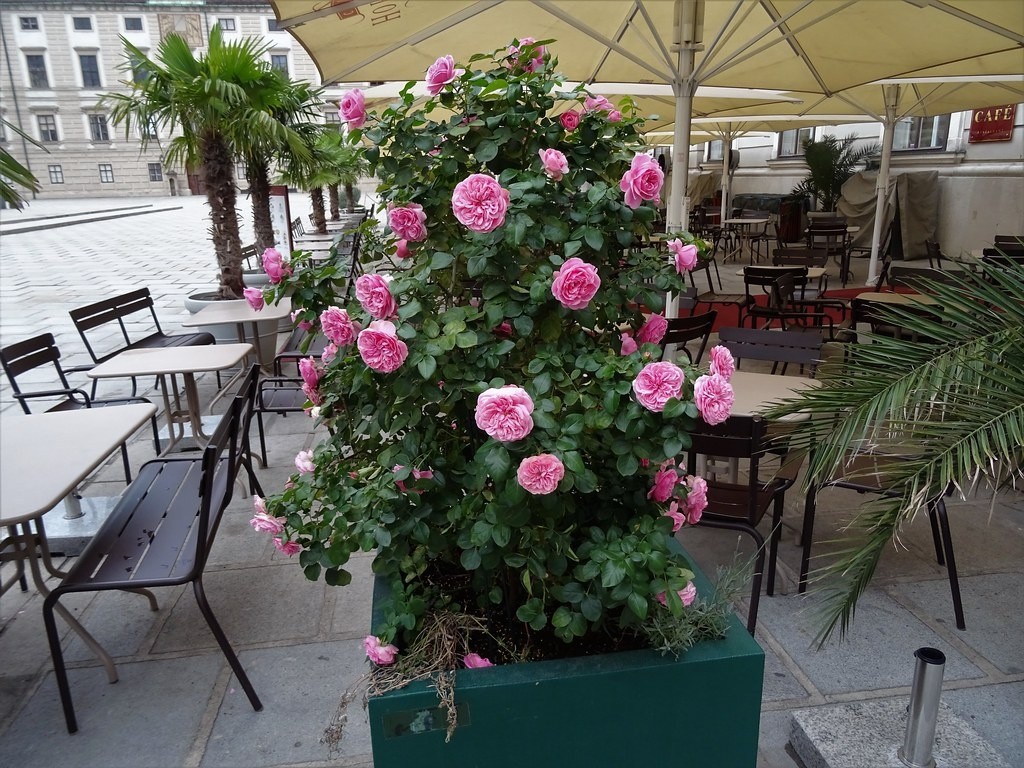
[269, 0, 1024, 365]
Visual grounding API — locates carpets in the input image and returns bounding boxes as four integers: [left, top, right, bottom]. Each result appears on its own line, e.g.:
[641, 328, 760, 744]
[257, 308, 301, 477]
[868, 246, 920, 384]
[620, 287, 921, 337]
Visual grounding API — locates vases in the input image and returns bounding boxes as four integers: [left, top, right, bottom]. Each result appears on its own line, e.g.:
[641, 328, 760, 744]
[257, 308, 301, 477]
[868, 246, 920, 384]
[368, 536, 766, 768]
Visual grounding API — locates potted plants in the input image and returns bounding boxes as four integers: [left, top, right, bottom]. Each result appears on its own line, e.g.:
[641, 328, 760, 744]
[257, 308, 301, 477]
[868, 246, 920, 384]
[782, 129, 882, 242]
[92, 21, 365, 377]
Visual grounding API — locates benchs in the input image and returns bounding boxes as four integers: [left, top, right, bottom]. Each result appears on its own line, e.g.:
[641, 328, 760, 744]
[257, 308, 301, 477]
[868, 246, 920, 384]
[42, 362, 264, 735]
[772, 215, 856, 288]
[69, 287, 222, 397]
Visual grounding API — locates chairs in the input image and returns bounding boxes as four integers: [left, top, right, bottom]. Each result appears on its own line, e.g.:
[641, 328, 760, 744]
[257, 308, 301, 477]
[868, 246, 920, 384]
[340, 246, 361, 281]
[717, 327, 825, 377]
[344, 203, 375, 248]
[770, 273, 858, 380]
[926, 240, 980, 283]
[0, 332, 142, 476]
[291, 221, 297, 239]
[982, 235, 1024, 320]
[273, 327, 332, 418]
[308, 212, 315, 225]
[650, 209, 769, 295]
[257, 354, 345, 468]
[242, 245, 259, 270]
[334, 279, 354, 306]
[687, 257, 757, 372]
[295, 216, 305, 236]
[799, 389, 967, 631]
[742, 266, 809, 345]
[337, 232, 363, 271]
[682, 414, 808, 638]
[888, 266, 966, 293]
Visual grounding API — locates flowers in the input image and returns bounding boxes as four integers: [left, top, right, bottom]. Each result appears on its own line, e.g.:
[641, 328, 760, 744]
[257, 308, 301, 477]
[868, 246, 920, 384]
[235, 37, 766, 668]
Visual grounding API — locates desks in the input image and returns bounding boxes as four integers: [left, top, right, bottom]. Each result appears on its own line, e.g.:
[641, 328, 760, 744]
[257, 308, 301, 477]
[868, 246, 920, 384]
[722, 219, 770, 266]
[87, 343, 264, 499]
[640, 236, 665, 251]
[1, 402, 159, 684]
[804, 226, 861, 233]
[182, 296, 293, 430]
[293, 212, 367, 259]
[700, 370, 822, 484]
[735, 265, 827, 290]
[695, 369, 823, 597]
[689, 213, 720, 216]
[845, 292, 974, 314]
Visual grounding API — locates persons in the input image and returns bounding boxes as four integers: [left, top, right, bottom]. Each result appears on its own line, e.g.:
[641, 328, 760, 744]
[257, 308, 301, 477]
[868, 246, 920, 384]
[657, 154, 667, 171]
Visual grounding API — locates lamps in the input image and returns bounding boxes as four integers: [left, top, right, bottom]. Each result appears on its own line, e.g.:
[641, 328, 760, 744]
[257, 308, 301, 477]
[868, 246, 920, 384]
[159, 155, 165, 162]
